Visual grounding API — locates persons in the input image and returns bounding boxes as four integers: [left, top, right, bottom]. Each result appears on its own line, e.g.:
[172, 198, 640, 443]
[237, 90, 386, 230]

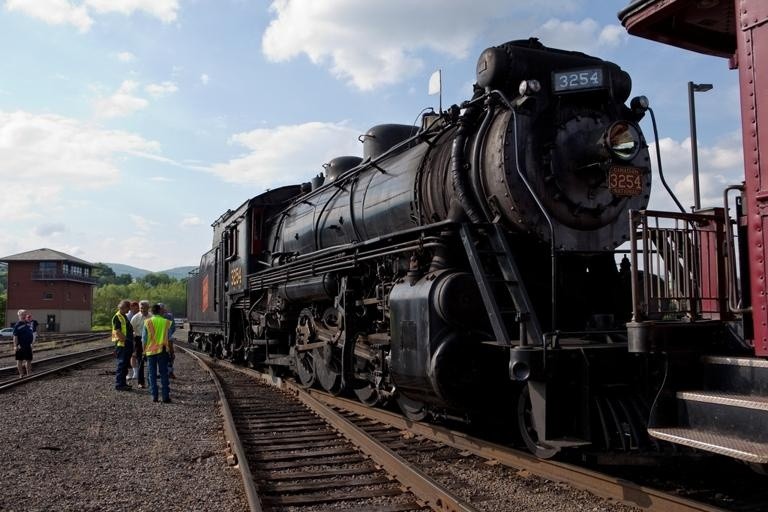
[110, 297, 176, 403]
[12, 309, 33, 377]
[46, 317, 57, 332]
[26, 314, 38, 346]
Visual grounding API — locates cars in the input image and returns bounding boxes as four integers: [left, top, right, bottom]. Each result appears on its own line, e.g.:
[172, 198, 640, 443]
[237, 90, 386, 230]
[0, 327, 15, 338]
[174, 319, 184, 329]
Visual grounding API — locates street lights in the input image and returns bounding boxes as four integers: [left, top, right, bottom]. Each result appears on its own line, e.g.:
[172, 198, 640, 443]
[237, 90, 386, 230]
[687, 81, 713, 212]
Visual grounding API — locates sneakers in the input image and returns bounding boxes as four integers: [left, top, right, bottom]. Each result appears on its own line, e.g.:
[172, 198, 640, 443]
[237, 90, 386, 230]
[114, 372, 177, 404]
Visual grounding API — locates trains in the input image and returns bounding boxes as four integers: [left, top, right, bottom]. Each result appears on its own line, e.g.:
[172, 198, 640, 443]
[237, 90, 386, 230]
[187, 36, 682, 467]
